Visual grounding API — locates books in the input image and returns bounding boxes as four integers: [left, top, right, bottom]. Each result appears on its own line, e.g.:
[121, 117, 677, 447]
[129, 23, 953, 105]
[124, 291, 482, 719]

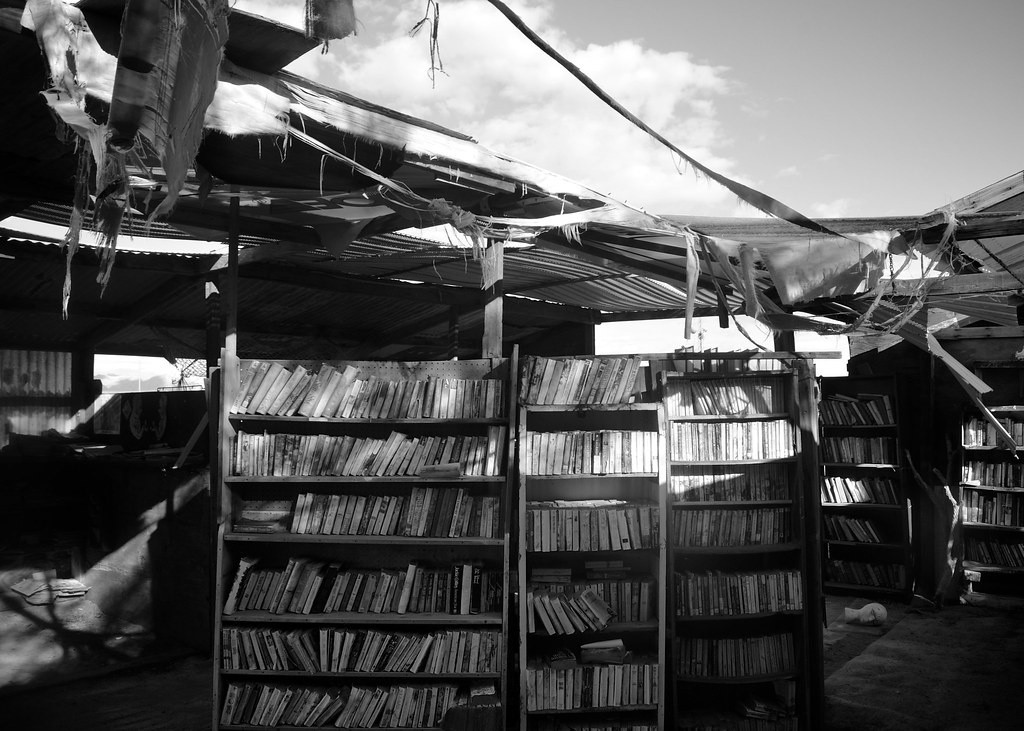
[819, 391, 911, 589]
[687, 682, 800, 731]
[220, 680, 503, 730]
[667, 377, 788, 417]
[230, 359, 507, 419]
[11, 570, 92, 606]
[526, 558, 655, 633]
[526, 430, 659, 475]
[672, 466, 796, 501]
[526, 498, 660, 551]
[567, 722, 658, 731]
[230, 424, 507, 475]
[222, 625, 503, 674]
[677, 628, 797, 678]
[0, 348, 72, 451]
[673, 507, 801, 547]
[961, 418, 1024, 568]
[234, 486, 504, 538]
[223, 556, 503, 614]
[526, 638, 660, 712]
[671, 419, 797, 462]
[519, 354, 642, 405]
[674, 568, 803, 616]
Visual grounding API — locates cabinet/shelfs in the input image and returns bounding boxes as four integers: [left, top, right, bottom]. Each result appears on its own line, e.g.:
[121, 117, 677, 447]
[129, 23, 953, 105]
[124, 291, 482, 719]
[210, 344, 1024, 731]
